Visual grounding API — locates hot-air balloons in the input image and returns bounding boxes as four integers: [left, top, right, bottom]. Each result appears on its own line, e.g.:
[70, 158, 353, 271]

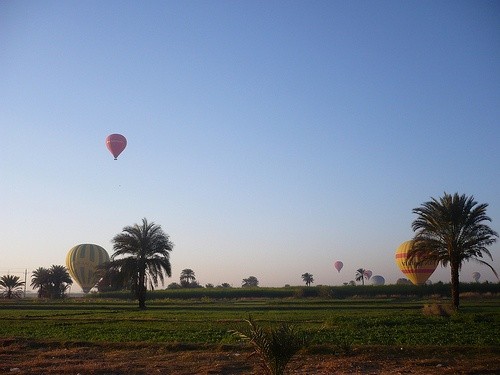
[66, 244, 110, 294]
[335, 261, 343, 272]
[365, 270, 372, 280]
[396, 240, 440, 286]
[472, 272, 481, 282]
[106, 134, 127, 160]
